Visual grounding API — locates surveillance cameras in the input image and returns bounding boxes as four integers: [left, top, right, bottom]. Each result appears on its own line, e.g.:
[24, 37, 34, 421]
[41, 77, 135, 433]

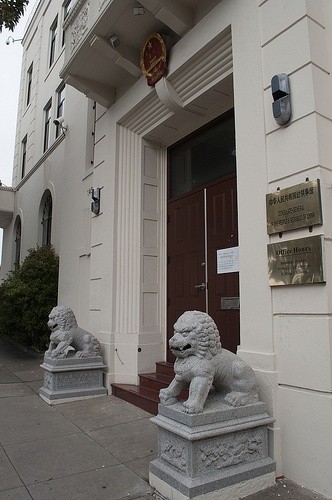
[53, 116, 64, 126]
